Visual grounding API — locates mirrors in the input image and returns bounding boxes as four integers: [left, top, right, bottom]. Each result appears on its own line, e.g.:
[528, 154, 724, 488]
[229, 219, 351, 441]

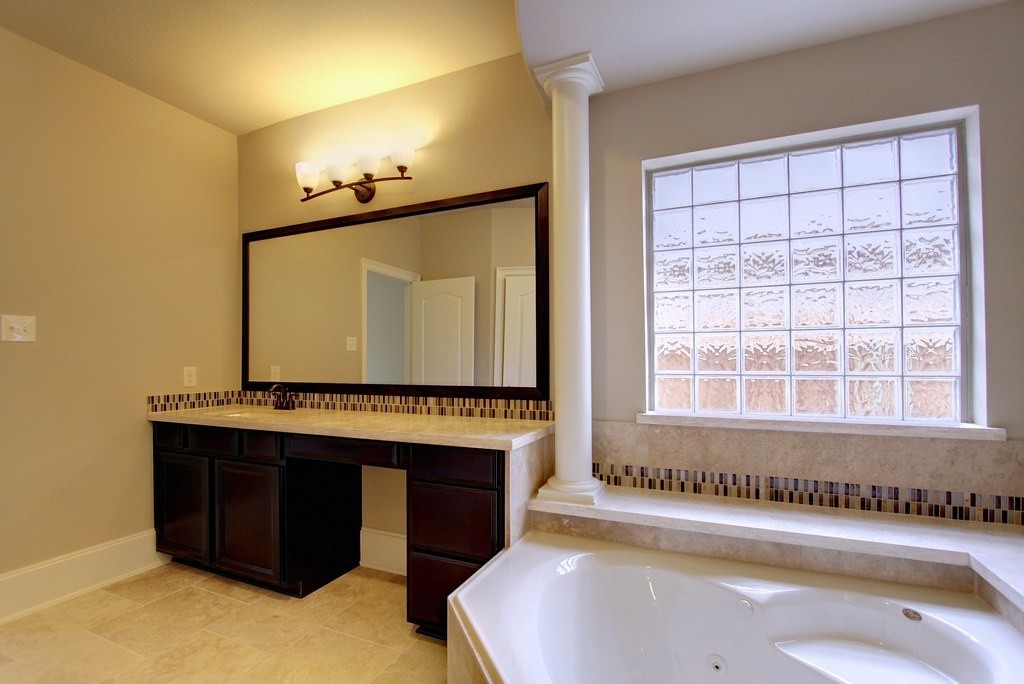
[242, 182, 550, 401]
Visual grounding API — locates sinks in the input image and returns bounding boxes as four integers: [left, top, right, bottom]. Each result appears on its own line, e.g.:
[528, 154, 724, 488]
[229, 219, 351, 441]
[205, 409, 285, 421]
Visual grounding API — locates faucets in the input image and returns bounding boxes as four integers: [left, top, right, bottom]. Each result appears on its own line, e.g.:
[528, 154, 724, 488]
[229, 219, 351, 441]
[268, 384, 286, 405]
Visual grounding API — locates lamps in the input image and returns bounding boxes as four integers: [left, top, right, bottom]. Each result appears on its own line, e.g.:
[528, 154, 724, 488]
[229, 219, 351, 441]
[294, 141, 413, 204]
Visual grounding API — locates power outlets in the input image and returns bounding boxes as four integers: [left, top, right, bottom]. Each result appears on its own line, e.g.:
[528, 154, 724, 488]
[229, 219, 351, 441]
[270, 366, 280, 381]
[183, 367, 197, 387]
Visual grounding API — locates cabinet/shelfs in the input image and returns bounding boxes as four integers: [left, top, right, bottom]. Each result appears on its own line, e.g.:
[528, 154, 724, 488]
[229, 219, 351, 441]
[152, 420, 505, 641]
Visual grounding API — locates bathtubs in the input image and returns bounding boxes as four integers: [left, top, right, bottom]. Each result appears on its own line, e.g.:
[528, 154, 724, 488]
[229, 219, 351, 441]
[447, 528, 1024, 684]
